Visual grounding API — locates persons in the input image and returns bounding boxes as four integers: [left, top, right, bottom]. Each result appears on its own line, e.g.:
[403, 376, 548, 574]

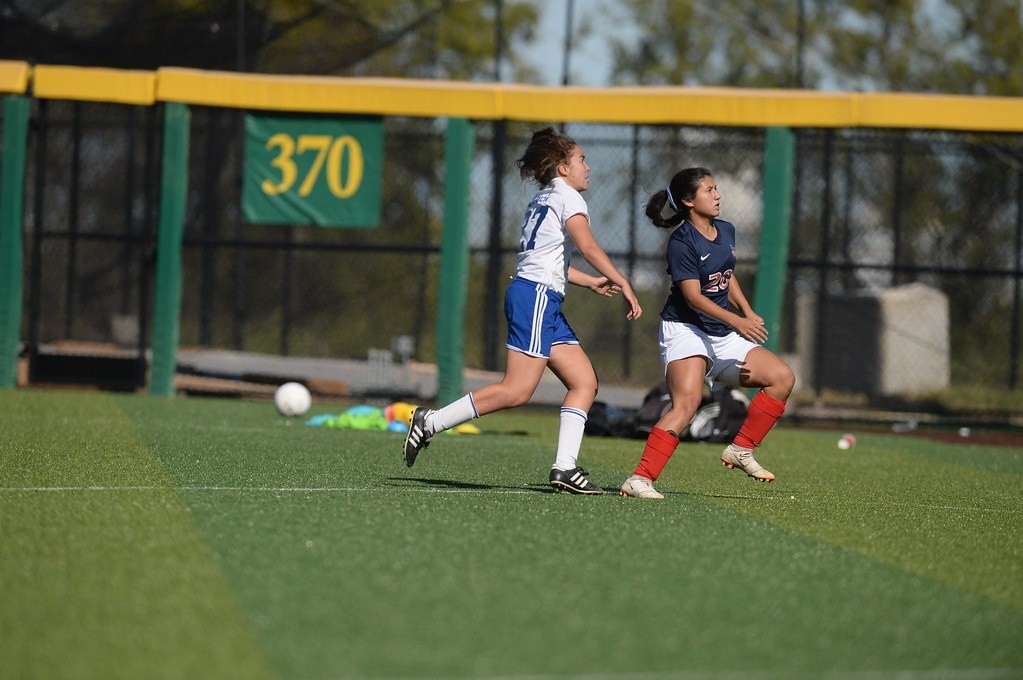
[403, 126, 644, 496]
[616, 167, 796, 500]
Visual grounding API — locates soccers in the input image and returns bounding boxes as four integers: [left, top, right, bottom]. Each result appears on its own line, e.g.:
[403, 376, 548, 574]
[273, 379, 313, 418]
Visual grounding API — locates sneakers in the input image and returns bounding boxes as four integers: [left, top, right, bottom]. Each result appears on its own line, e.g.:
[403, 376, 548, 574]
[620, 477, 664, 499]
[402, 407, 436, 467]
[549, 466, 603, 495]
[720, 443, 775, 482]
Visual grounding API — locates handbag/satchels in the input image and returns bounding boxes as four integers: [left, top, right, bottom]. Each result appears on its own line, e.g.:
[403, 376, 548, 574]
[628, 379, 749, 441]
